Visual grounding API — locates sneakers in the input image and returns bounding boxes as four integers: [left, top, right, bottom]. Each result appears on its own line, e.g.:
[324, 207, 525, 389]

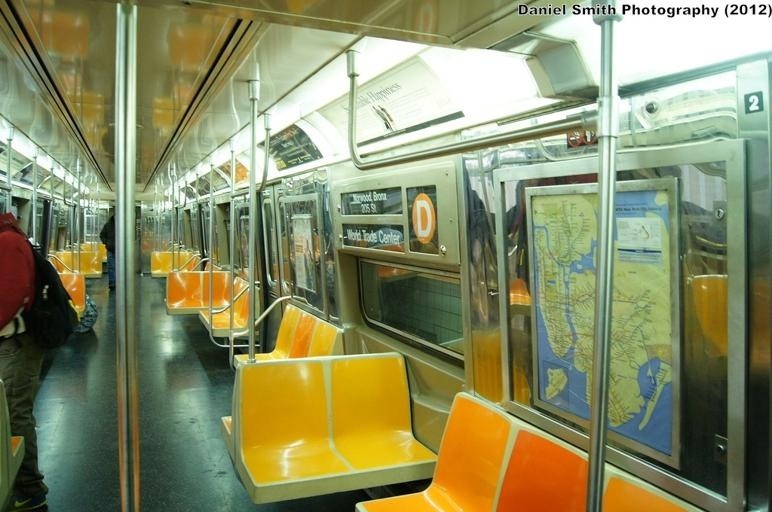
[7, 497, 48, 511]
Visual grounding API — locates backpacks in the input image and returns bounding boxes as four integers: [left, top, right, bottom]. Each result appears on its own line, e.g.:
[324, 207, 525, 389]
[25, 234, 78, 350]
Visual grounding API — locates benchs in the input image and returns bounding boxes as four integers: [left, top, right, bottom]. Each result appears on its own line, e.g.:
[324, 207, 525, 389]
[46, 236, 106, 318]
[24, 2, 232, 187]
[0, 380, 27, 491]
[0, 379, 11, 509]
[685, 272, 772, 387]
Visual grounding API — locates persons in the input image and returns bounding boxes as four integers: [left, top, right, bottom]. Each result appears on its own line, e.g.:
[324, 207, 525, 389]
[99, 113, 116, 163]
[98, 214, 116, 290]
[0, 212, 51, 512]
[364, 224, 418, 329]
[470, 164, 600, 296]
[656, 166, 728, 257]
